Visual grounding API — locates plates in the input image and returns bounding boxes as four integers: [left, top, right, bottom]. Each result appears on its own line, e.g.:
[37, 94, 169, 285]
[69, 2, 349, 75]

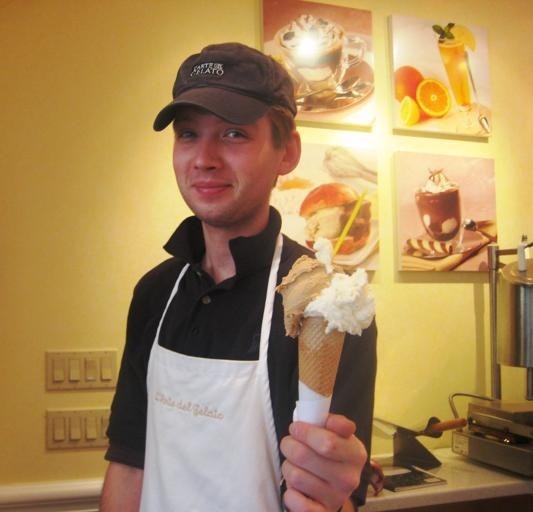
[264, 52, 374, 114]
[320, 215, 380, 268]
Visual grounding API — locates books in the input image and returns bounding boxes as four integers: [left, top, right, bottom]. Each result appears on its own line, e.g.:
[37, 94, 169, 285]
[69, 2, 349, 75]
[376, 462, 449, 493]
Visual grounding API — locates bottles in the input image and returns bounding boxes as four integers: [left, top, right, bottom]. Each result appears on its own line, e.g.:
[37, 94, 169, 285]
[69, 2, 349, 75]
[516, 234, 529, 271]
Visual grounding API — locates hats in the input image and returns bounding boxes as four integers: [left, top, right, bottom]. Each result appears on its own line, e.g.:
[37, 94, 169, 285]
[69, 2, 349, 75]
[153, 42, 295, 131]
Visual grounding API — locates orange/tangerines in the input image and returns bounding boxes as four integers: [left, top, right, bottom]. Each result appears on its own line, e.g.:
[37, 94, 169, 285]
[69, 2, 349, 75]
[393, 66, 450, 126]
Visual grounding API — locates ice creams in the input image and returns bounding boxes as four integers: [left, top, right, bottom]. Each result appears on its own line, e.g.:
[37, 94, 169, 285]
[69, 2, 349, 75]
[416, 169, 460, 243]
[273, 237, 376, 425]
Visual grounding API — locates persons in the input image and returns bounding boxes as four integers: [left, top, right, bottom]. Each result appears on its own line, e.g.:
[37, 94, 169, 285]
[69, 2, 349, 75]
[96, 41, 379, 512]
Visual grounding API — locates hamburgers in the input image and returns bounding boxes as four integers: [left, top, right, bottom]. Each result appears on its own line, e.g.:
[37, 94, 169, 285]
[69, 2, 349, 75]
[300, 183, 372, 255]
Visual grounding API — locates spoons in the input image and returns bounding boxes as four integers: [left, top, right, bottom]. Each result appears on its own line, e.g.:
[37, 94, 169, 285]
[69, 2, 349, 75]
[464, 218, 497, 245]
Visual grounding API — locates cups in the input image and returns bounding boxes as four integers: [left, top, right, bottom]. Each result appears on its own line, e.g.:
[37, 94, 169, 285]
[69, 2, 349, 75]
[437, 39, 480, 112]
[413, 188, 463, 244]
[273, 17, 366, 95]
